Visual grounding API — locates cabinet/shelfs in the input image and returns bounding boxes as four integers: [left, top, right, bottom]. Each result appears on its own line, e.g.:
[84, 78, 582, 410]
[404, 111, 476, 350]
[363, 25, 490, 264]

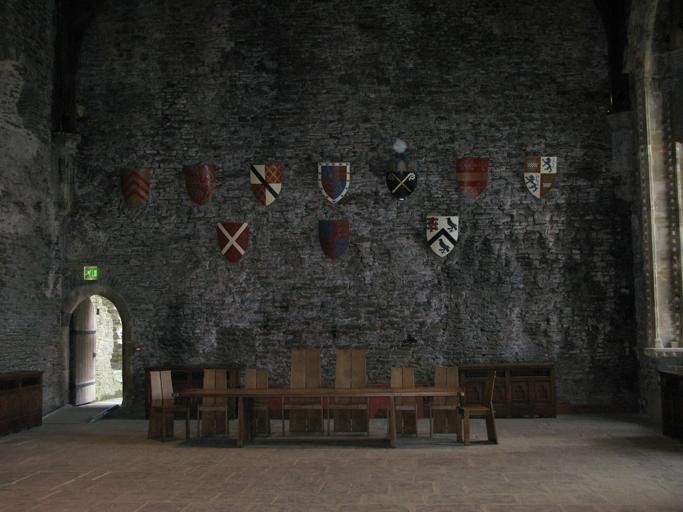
[0, 368, 44, 439]
[460, 360, 558, 419]
